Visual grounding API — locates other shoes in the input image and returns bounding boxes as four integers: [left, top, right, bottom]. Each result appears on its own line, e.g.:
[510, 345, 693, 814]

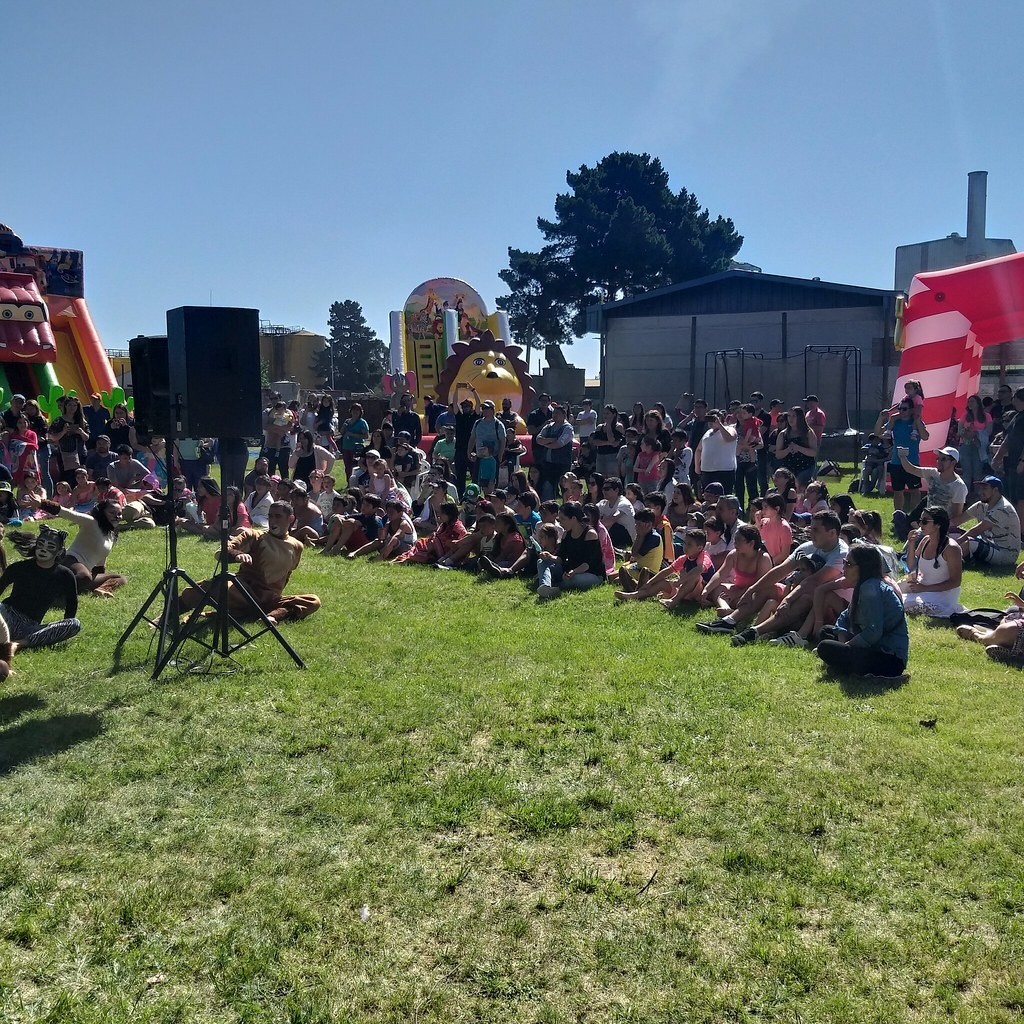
[956, 625, 1017, 664]
[434, 556, 656, 598]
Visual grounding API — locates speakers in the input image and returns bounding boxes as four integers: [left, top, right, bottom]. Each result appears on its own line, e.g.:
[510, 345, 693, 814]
[129, 335, 170, 448]
[166, 306, 263, 441]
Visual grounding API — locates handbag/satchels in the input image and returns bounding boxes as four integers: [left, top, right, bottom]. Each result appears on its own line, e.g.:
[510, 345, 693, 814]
[61, 450, 80, 471]
[200, 447, 214, 464]
[817, 459, 874, 492]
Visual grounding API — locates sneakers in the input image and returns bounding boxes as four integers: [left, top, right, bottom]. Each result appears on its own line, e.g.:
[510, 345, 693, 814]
[696, 617, 734, 635]
[770, 631, 809, 647]
[732, 627, 757, 646]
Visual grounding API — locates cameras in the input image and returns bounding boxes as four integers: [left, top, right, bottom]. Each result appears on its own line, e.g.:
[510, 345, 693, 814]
[901, 448, 909, 456]
[306, 402, 313, 408]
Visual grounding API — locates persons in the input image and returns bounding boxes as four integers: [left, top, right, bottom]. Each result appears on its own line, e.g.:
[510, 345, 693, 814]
[150, 500, 321, 624]
[0, 381, 1023, 681]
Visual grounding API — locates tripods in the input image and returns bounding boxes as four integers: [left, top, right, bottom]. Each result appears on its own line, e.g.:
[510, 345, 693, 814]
[117, 441, 308, 682]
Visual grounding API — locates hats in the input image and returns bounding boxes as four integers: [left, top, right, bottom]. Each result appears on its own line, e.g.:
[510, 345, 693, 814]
[973, 475, 1003, 490]
[267, 384, 820, 457]
[795, 550, 826, 572]
[464, 485, 508, 500]
[704, 483, 724, 497]
[0, 392, 112, 514]
[933, 447, 959, 463]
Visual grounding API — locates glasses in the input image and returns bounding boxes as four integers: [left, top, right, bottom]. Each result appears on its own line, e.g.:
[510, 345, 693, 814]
[843, 558, 857, 568]
[366, 455, 375, 460]
[918, 517, 934, 525]
[898, 406, 910, 411]
[587, 481, 614, 493]
[936, 457, 951, 463]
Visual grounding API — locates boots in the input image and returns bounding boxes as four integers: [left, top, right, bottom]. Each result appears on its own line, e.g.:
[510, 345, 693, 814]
[258, 607, 288, 625]
[149, 596, 189, 629]
[94, 578, 121, 597]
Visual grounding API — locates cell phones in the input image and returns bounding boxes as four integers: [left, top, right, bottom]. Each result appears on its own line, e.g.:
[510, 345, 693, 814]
[458, 383, 467, 388]
[704, 415, 716, 423]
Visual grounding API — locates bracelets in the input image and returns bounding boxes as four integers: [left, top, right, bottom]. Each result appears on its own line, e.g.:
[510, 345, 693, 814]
[815, 621, 823, 624]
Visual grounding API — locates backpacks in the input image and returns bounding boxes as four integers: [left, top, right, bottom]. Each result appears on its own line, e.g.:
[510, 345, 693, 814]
[950, 608, 1008, 630]
